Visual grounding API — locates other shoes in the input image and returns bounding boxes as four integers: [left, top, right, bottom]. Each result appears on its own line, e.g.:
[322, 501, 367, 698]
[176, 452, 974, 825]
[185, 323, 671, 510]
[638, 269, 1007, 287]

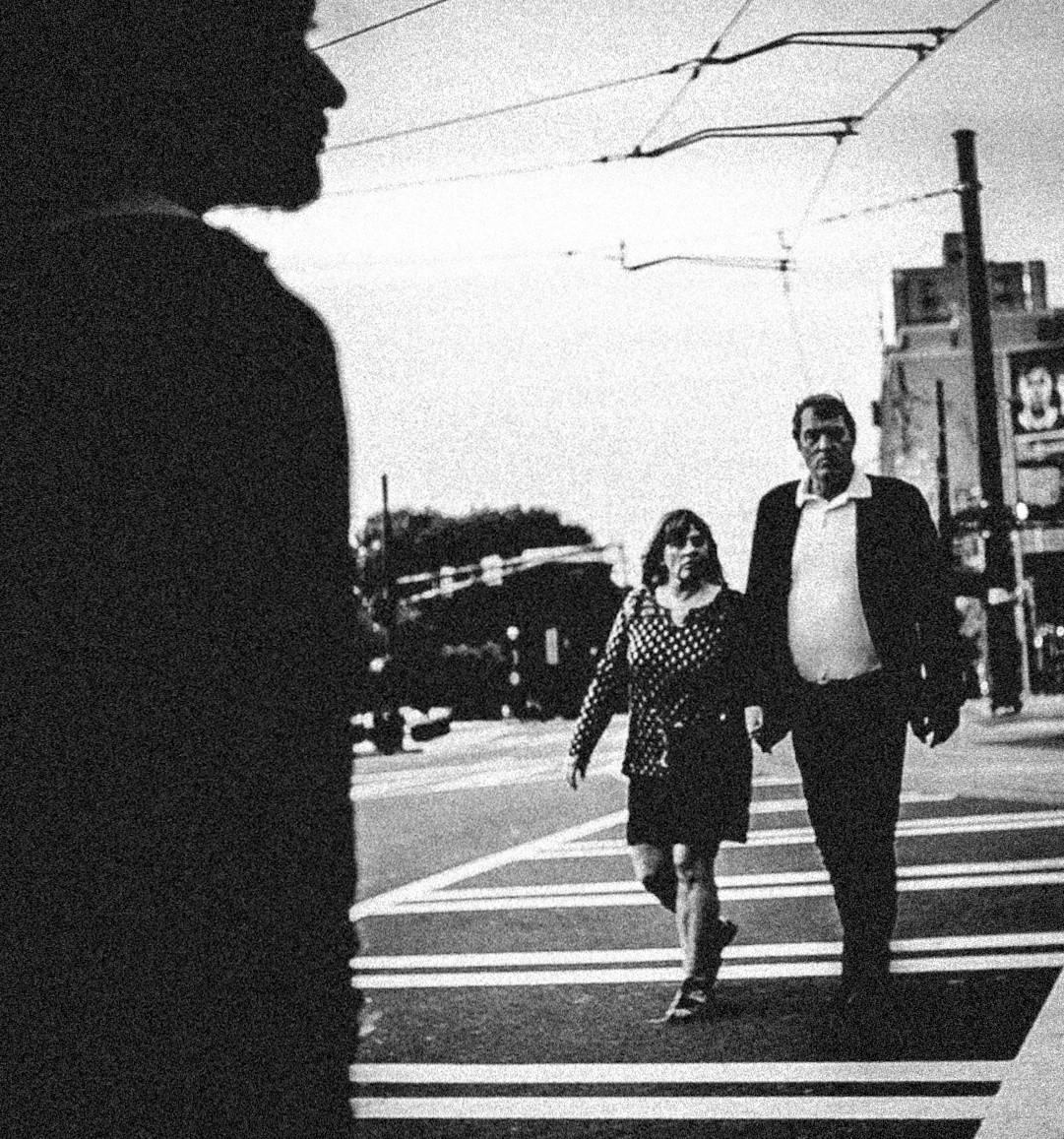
[709, 918, 737, 977]
[666, 976, 712, 1025]
[832, 955, 877, 1013]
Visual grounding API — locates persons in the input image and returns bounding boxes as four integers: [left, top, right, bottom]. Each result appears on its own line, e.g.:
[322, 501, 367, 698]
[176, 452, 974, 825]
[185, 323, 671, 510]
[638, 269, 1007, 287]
[742, 391, 961, 1011]
[563, 508, 767, 1025]
[2, 2, 368, 1136]
